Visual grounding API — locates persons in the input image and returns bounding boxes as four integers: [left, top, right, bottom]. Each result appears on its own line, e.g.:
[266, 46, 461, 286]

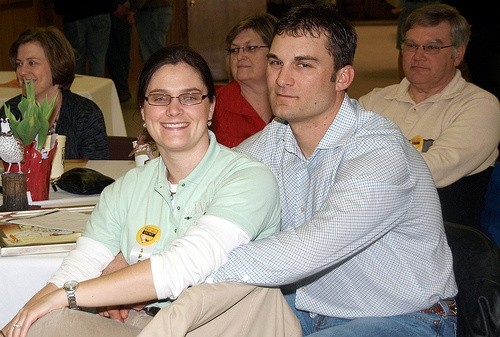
[94, 5, 458, 337]
[0, 25, 110, 159]
[0, 46, 282, 337]
[56, 0, 174, 103]
[208, 13, 282, 149]
[358, 4, 500, 232]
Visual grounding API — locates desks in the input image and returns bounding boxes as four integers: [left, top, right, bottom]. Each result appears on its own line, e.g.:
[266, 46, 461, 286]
[0, 70, 127, 138]
[0, 159, 138, 210]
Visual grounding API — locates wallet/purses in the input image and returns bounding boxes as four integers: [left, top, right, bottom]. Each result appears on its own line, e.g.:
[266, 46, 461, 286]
[51, 168, 116, 195]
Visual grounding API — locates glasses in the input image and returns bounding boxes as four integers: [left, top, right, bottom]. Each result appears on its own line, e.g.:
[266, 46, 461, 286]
[224, 45, 270, 56]
[401, 40, 455, 55]
[143, 93, 209, 106]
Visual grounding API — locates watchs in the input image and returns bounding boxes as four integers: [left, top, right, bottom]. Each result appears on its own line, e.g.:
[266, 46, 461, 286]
[64, 280, 80, 310]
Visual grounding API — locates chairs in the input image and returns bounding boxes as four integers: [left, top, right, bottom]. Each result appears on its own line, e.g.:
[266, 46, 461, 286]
[443, 219, 498, 337]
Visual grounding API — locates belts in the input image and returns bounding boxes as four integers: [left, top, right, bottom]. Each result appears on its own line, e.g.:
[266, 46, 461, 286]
[417, 301, 458, 316]
[144, 306, 160, 318]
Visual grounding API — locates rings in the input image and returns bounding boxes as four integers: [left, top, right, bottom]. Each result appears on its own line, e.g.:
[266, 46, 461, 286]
[13, 323, 22, 328]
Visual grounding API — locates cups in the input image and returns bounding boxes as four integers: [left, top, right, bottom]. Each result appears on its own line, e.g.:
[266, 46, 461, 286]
[132, 140, 159, 168]
[50, 134, 68, 180]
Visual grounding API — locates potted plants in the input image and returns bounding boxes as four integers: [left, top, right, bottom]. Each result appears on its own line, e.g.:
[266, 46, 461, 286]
[1, 76, 61, 201]
